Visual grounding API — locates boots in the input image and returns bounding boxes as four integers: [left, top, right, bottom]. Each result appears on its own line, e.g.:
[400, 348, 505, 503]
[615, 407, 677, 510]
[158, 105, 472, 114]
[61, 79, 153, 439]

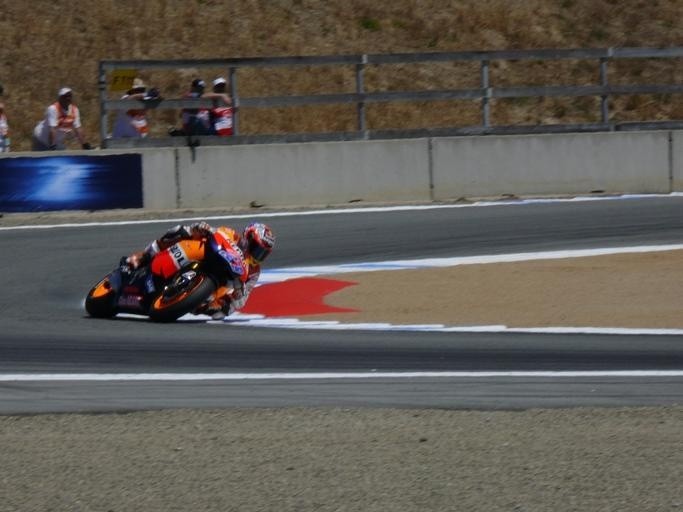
[126, 239, 160, 271]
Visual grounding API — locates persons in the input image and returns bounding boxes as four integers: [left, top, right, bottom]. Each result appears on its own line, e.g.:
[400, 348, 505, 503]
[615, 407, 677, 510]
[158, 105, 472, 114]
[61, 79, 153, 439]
[212, 76, 241, 136]
[180, 78, 232, 129]
[32, 87, 95, 151]
[119, 77, 150, 138]
[0, 85, 11, 153]
[119, 220, 275, 320]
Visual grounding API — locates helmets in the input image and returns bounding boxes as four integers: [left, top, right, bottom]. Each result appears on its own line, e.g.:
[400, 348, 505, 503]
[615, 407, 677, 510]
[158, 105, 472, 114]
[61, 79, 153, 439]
[242, 223, 275, 263]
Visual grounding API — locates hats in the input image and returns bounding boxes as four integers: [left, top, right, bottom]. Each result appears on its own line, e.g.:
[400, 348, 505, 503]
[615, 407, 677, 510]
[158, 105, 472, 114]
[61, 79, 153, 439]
[191, 78, 206, 88]
[126, 79, 147, 94]
[59, 88, 72, 96]
[213, 77, 228, 87]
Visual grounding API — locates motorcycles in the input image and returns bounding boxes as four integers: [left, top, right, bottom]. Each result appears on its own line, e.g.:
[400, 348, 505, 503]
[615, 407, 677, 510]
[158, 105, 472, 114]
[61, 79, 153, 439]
[85, 221, 250, 322]
